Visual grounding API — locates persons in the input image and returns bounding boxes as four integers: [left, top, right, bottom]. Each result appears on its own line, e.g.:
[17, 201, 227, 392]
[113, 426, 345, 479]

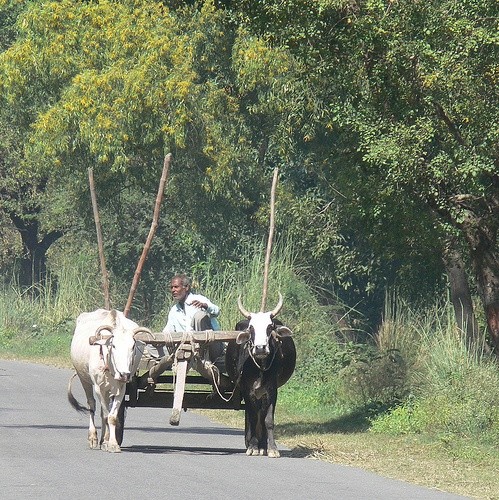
[147, 274, 223, 367]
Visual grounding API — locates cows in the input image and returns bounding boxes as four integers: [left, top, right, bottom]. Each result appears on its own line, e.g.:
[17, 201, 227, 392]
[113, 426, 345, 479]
[67, 309, 156, 454]
[225, 290, 297, 459]
